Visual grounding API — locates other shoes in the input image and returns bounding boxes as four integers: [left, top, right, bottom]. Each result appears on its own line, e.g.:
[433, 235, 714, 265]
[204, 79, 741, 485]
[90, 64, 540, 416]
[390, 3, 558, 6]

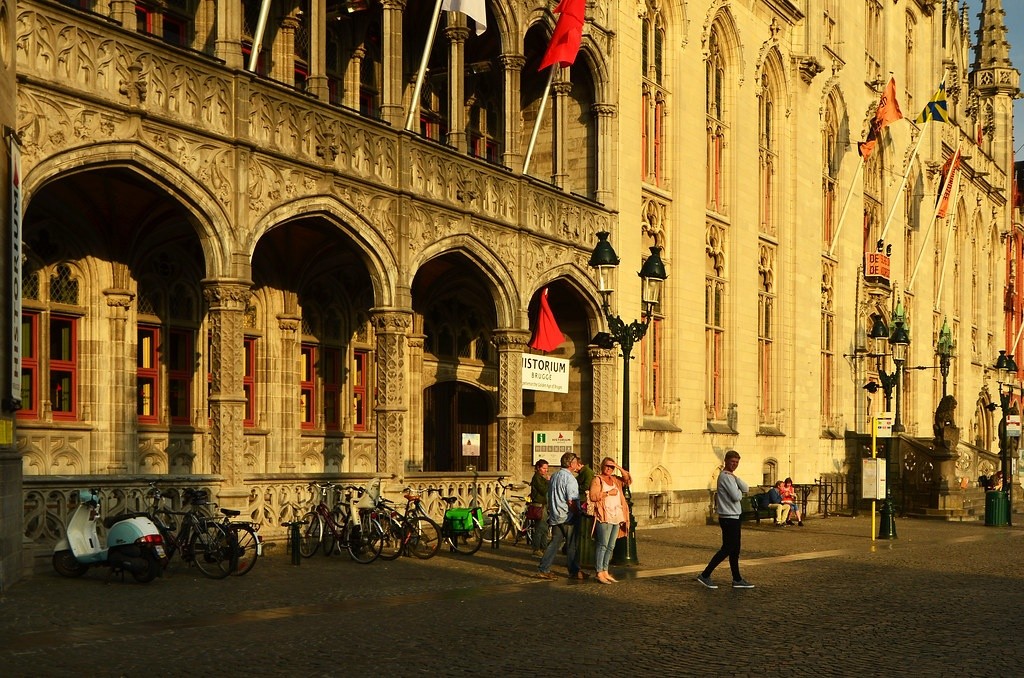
[798, 521, 804, 526]
[782, 523, 791, 527]
[568, 571, 589, 580]
[531, 550, 544, 559]
[787, 520, 795, 526]
[537, 571, 558, 581]
[776, 523, 782, 528]
[595, 571, 620, 585]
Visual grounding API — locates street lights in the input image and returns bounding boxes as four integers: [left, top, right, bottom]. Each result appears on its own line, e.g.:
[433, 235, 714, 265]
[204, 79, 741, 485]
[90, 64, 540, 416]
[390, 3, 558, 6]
[866, 316, 915, 538]
[989, 350, 1020, 524]
[588, 229, 664, 563]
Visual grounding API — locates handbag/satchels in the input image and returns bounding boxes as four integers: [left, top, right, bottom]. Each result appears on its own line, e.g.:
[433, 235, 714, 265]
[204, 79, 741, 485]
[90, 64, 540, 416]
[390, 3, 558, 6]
[524, 502, 543, 520]
[445, 506, 484, 533]
[584, 476, 602, 516]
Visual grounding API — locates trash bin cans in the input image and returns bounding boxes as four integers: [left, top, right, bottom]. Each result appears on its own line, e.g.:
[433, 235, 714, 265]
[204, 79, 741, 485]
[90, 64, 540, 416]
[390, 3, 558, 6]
[984, 490, 1009, 527]
[578, 514, 598, 569]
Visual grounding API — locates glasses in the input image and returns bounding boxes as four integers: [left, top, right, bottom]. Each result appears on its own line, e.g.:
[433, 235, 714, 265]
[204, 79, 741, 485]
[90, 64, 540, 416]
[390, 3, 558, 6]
[571, 453, 576, 462]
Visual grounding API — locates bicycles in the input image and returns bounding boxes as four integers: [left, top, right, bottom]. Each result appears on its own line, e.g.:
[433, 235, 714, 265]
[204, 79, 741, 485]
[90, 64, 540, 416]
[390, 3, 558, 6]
[298, 480, 443, 565]
[477, 475, 557, 549]
[402, 487, 485, 557]
[142, 481, 265, 579]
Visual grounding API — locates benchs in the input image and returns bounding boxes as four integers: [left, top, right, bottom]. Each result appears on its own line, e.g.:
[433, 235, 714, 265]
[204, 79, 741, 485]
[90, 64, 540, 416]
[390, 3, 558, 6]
[752, 493, 777, 526]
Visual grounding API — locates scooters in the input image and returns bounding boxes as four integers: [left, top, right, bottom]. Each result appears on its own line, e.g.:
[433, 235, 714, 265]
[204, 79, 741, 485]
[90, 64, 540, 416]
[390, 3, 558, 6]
[48, 486, 173, 585]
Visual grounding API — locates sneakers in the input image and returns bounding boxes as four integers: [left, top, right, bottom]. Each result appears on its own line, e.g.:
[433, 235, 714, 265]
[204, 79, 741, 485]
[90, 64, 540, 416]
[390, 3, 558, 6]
[696, 574, 719, 589]
[732, 578, 754, 588]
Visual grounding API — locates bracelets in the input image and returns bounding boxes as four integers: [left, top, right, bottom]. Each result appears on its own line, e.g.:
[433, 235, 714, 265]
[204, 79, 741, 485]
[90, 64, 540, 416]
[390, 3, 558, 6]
[606, 490, 609, 495]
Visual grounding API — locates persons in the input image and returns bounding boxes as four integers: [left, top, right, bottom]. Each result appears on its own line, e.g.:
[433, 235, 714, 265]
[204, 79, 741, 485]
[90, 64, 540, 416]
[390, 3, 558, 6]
[697, 450, 755, 588]
[590, 457, 632, 584]
[767, 480, 791, 527]
[530, 459, 551, 557]
[778, 477, 804, 526]
[561, 457, 596, 555]
[537, 452, 589, 580]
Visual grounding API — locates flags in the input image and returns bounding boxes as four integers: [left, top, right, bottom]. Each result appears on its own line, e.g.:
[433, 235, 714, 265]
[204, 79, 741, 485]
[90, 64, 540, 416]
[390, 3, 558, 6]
[531, 293, 565, 352]
[935, 147, 961, 218]
[538, 0, 586, 71]
[914, 79, 949, 124]
[440, 0, 487, 36]
[858, 77, 904, 162]
